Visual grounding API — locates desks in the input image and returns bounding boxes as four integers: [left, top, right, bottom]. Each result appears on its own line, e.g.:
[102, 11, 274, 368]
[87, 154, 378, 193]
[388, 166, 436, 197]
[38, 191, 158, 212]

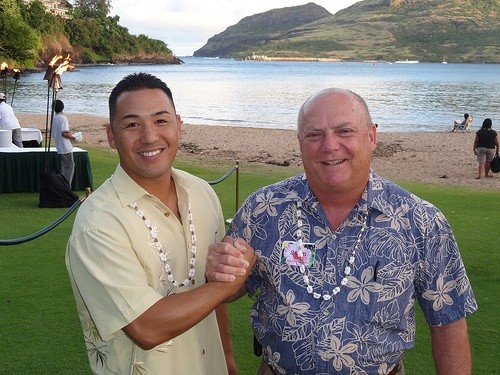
[0, 147, 93, 192]
[20, 128, 44, 147]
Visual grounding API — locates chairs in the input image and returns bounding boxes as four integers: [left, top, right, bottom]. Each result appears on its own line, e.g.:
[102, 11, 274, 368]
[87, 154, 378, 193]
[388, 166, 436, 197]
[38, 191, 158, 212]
[455, 114, 473, 133]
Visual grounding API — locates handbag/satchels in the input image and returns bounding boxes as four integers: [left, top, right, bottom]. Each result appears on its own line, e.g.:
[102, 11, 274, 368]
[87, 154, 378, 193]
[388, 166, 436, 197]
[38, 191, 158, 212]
[487, 151, 500, 173]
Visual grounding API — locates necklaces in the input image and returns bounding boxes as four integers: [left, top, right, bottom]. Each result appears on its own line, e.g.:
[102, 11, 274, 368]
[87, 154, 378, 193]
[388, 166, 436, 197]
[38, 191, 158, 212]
[129, 201, 196, 288]
[296, 202, 369, 300]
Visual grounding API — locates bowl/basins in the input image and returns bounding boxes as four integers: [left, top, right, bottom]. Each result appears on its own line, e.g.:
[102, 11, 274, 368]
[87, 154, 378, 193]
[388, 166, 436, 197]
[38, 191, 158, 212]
[72, 132, 82, 143]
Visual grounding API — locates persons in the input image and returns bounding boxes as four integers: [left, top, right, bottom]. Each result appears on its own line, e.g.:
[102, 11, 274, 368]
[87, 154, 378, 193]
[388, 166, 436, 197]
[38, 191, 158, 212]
[0, 93, 24, 148]
[51, 100, 77, 191]
[450, 113, 469, 132]
[203, 88, 478, 375]
[65, 73, 257, 375]
[473, 118, 499, 179]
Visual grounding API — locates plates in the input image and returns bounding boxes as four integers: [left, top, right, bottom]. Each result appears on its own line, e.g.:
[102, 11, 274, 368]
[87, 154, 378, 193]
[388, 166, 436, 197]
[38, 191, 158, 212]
[0, 130, 12, 146]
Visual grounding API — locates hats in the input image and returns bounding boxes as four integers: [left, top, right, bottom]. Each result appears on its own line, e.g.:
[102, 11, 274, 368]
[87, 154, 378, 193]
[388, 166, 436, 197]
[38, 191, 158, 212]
[0, 93, 5, 99]
